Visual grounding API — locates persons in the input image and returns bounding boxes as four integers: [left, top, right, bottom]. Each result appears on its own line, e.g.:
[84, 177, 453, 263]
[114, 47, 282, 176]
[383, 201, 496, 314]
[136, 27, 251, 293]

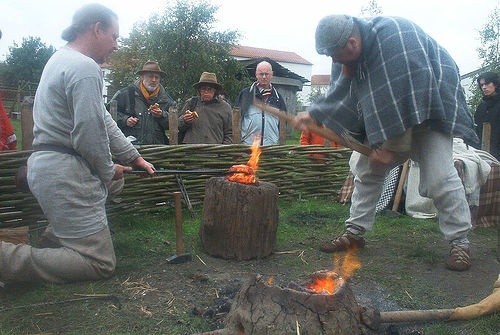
[300, 98, 342, 160]
[293, 14, 480, 272]
[473, 72, 500, 159]
[177, 72, 233, 144]
[0, 3, 157, 286]
[236, 61, 287, 146]
[0, 94, 18, 150]
[105, 62, 177, 145]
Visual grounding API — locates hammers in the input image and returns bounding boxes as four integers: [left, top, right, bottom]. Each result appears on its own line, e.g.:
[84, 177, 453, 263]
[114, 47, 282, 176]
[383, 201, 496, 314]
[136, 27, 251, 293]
[383, 157, 411, 217]
[241, 91, 396, 166]
[166, 191, 192, 264]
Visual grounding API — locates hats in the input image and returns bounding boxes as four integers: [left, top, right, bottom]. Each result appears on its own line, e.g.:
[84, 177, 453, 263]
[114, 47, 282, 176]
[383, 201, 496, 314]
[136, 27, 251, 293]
[135, 62, 168, 78]
[192, 71, 223, 89]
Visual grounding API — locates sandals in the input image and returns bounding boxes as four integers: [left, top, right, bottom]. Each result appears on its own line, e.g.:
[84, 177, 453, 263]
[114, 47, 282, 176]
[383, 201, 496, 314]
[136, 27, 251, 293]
[449, 247, 473, 268]
[321, 232, 368, 250]
